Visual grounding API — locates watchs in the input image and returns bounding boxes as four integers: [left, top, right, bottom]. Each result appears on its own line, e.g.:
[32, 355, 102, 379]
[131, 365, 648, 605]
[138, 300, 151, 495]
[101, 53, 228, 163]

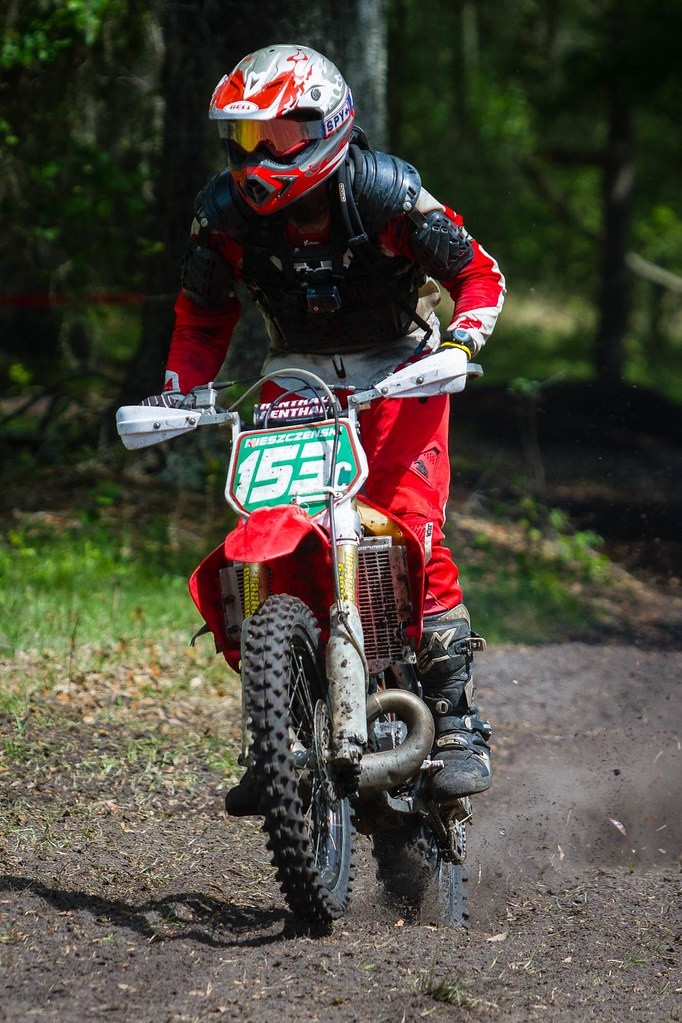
[442, 328, 475, 355]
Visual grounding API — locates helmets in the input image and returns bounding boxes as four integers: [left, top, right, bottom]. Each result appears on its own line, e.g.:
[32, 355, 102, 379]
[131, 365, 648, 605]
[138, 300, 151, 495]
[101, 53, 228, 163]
[209, 44, 356, 214]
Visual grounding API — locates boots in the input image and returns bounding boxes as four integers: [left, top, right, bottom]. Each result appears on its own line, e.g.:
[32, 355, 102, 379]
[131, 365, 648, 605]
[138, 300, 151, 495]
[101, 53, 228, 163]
[424, 604, 490, 796]
[224, 765, 263, 817]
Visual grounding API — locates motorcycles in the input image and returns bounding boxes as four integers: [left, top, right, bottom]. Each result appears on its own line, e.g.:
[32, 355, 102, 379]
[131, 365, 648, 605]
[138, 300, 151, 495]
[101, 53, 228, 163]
[113, 347, 482, 939]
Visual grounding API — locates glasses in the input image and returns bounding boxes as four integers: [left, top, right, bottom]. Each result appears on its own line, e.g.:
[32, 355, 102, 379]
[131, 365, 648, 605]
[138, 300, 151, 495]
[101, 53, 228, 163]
[217, 118, 324, 157]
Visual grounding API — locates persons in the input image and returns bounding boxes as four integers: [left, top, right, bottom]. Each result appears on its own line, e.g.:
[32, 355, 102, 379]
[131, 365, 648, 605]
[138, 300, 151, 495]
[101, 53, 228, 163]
[139, 42, 508, 818]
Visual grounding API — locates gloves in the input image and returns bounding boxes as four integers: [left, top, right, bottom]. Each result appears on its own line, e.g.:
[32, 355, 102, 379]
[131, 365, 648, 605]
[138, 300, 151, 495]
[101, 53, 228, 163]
[139, 395, 191, 411]
[419, 342, 472, 403]
